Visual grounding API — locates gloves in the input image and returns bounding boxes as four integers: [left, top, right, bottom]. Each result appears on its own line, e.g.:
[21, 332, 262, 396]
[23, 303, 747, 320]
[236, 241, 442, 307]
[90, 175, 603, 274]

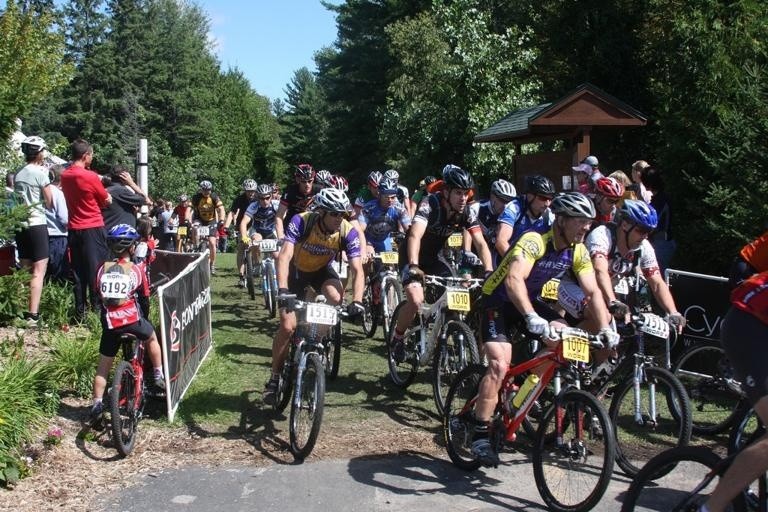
[241, 236, 252, 244]
[669, 312, 686, 326]
[609, 301, 629, 316]
[522, 313, 550, 338]
[278, 287, 297, 314]
[347, 301, 365, 321]
[464, 250, 478, 265]
[408, 263, 425, 281]
[598, 328, 620, 348]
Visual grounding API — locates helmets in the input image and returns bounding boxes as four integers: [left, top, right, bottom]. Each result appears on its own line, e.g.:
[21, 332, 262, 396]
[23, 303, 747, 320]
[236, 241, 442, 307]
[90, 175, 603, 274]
[200, 180, 212, 190]
[316, 188, 350, 212]
[492, 179, 517, 201]
[527, 175, 556, 195]
[425, 175, 437, 185]
[596, 177, 626, 197]
[442, 164, 475, 190]
[22, 136, 49, 152]
[315, 169, 332, 182]
[323, 175, 349, 192]
[620, 198, 658, 229]
[384, 170, 399, 180]
[378, 177, 398, 194]
[243, 179, 258, 192]
[181, 194, 188, 202]
[368, 171, 383, 188]
[551, 192, 596, 219]
[257, 184, 272, 196]
[295, 164, 315, 180]
[106, 223, 141, 253]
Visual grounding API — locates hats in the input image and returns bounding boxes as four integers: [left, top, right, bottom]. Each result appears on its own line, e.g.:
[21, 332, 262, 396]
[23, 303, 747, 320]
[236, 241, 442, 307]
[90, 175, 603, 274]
[585, 156, 598, 165]
[572, 164, 593, 176]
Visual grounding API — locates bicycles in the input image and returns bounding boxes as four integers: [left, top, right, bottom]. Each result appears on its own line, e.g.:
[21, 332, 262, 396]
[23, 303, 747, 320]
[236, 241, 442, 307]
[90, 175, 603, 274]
[359, 234, 407, 335]
[728, 378, 768, 454]
[173, 223, 278, 320]
[617, 445, 768, 512]
[110, 274, 169, 456]
[386, 274, 488, 418]
[439, 323, 616, 510]
[272, 288, 364, 461]
[322, 307, 342, 381]
[664, 341, 752, 434]
[520, 303, 691, 482]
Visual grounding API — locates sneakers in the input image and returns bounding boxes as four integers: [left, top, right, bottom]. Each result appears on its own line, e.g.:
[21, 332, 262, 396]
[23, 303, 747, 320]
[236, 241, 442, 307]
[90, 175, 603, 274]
[263, 378, 279, 405]
[211, 265, 216, 275]
[391, 336, 405, 362]
[26, 317, 51, 330]
[91, 402, 104, 420]
[154, 379, 166, 397]
[470, 431, 500, 468]
[510, 401, 542, 416]
[15, 312, 25, 328]
[239, 280, 244, 286]
[582, 415, 603, 437]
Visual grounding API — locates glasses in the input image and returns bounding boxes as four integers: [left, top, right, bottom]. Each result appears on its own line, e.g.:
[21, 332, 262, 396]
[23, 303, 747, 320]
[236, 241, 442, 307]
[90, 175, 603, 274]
[258, 196, 271, 200]
[634, 224, 651, 235]
[329, 212, 346, 217]
[605, 198, 620, 205]
[575, 218, 592, 225]
[300, 180, 313, 183]
[537, 194, 554, 202]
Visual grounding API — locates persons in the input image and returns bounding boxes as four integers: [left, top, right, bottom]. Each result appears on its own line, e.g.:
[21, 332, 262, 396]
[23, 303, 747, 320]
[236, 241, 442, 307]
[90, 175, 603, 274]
[223, 163, 369, 405]
[6, 116, 151, 327]
[694, 235, 768, 512]
[350, 155, 686, 468]
[149, 180, 226, 274]
[92, 218, 166, 431]
